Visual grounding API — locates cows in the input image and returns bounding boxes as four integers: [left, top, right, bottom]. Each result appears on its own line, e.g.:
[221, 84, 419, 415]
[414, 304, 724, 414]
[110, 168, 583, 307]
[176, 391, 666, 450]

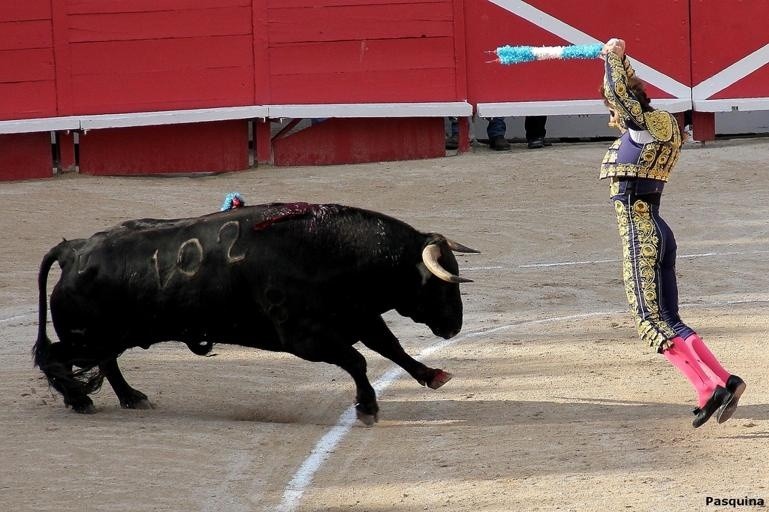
[33, 203, 481, 426]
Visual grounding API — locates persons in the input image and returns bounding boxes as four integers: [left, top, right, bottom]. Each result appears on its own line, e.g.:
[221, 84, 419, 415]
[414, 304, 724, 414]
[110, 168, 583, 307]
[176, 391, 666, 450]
[443, 117, 512, 151]
[597, 37, 746, 427]
[524, 116, 548, 150]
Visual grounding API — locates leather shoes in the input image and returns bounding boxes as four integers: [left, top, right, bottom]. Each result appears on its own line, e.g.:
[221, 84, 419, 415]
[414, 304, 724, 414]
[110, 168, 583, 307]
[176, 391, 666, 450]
[690, 385, 732, 429]
[714, 372, 748, 426]
